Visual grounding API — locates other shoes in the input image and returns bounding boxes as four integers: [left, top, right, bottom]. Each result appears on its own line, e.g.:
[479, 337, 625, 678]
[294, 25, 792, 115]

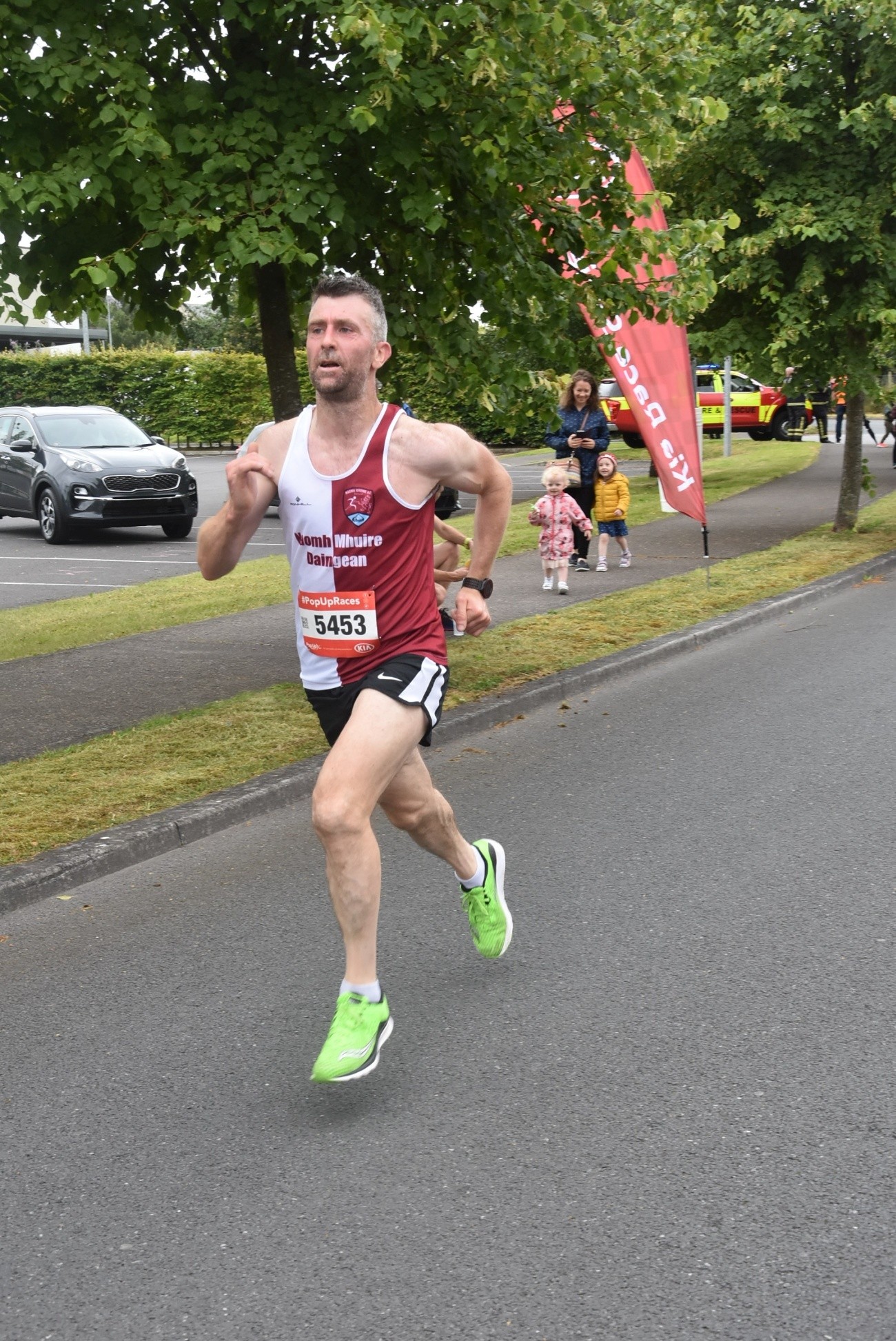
[822, 439, 834, 443]
[877, 442, 888, 448]
[836, 440, 840, 444]
[893, 463, 896, 469]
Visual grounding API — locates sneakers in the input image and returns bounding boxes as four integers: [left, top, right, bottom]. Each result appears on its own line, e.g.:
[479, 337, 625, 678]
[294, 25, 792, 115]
[458, 839, 514, 961]
[619, 552, 632, 567]
[575, 560, 590, 571]
[558, 583, 568, 595]
[543, 577, 554, 590]
[438, 606, 454, 631]
[567, 554, 578, 567]
[310, 992, 394, 1084]
[596, 558, 608, 571]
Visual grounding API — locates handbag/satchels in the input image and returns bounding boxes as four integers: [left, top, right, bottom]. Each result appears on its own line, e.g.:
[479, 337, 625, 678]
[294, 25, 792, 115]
[544, 456, 582, 486]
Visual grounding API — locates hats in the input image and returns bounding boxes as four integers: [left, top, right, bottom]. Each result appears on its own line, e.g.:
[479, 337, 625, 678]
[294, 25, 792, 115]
[597, 453, 618, 468]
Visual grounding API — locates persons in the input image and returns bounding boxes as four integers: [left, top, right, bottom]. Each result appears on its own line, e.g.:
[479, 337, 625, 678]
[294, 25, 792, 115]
[544, 369, 612, 571]
[527, 466, 595, 596]
[196, 277, 517, 1086]
[787, 371, 881, 447]
[884, 406, 896, 470]
[433, 484, 473, 631]
[594, 451, 632, 571]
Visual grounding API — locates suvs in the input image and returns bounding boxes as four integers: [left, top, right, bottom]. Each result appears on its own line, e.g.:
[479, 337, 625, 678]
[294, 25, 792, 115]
[0, 405, 199, 546]
[596, 362, 815, 448]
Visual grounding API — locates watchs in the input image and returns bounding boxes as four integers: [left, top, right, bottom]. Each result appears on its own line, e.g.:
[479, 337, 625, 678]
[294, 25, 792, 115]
[463, 538, 472, 548]
[461, 577, 493, 599]
